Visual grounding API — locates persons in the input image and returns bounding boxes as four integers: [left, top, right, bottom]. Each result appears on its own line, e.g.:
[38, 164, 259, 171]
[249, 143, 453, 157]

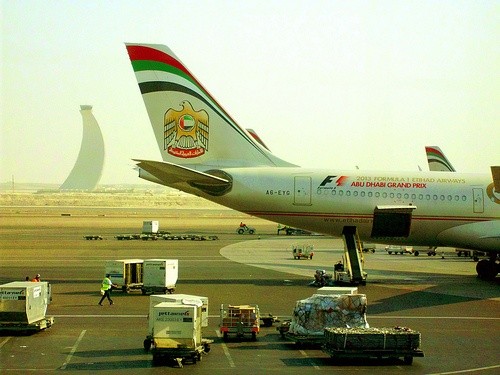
[314, 270, 326, 286]
[277, 224, 281, 235]
[32, 274, 41, 282]
[310, 250, 313, 259]
[335, 260, 343, 271]
[98, 274, 118, 306]
[240, 222, 247, 229]
[25, 277, 31, 282]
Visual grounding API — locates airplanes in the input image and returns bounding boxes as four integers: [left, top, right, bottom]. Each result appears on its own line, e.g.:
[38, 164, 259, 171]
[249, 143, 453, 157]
[125, 43, 500, 287]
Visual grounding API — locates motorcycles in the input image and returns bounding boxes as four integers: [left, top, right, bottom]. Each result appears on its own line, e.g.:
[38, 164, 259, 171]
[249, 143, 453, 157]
[235, 225, 256, 234]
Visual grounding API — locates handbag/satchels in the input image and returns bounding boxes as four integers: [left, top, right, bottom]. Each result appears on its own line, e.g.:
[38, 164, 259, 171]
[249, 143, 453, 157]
[100, 289, 105, 295]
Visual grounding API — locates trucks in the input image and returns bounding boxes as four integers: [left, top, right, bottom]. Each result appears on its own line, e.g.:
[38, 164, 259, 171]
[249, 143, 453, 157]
[145, 294, 211, 367]
[384, 244, 486, 261]
[292, 244, 314, 260]
[142, 220, 159, 240]
[0, 281, 55, 332]
[219, 304, 260, 342]
[105, 259, 179, 294]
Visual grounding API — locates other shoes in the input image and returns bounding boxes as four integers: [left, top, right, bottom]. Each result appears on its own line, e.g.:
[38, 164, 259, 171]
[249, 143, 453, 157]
[110, 303, 114, 305]
[99, 303, 103, 306]
